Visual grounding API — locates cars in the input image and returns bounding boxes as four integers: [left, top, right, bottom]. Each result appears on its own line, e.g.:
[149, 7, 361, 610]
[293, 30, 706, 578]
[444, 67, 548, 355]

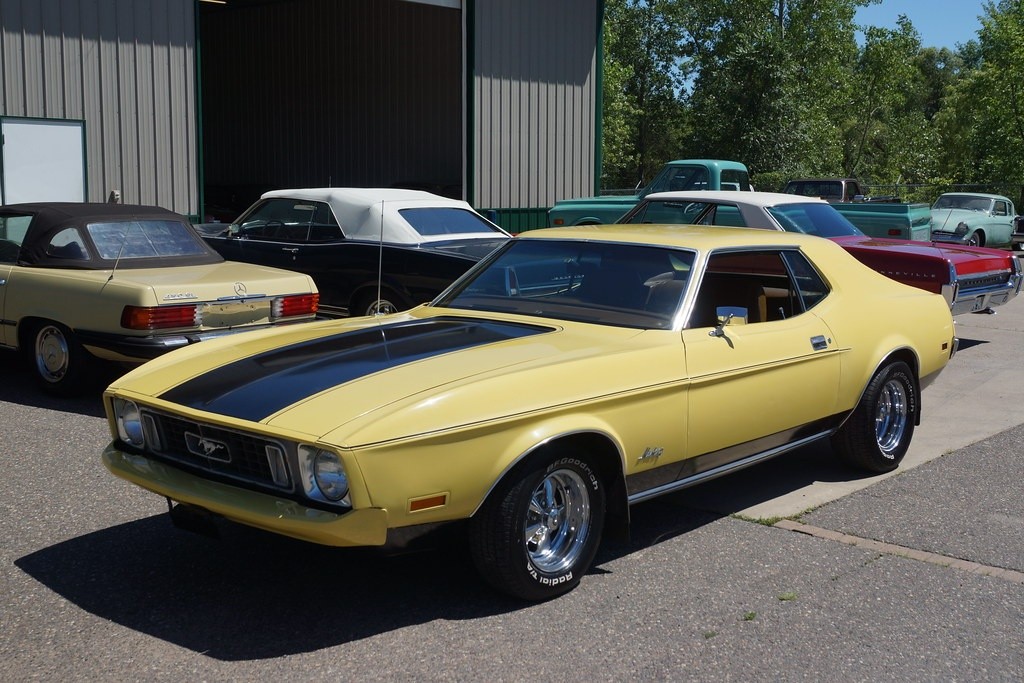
[604, 190, 1023, 320]
[931, 192, 1021, 248]
[102, 225, 958, 602]
[1, 202, 320, 397]
[190, 185, 514, 320]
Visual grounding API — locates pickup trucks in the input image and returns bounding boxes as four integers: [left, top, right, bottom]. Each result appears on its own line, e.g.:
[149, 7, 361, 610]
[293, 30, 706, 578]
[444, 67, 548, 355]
[781, 178, 901, 203]
[546, 157, 932, 244]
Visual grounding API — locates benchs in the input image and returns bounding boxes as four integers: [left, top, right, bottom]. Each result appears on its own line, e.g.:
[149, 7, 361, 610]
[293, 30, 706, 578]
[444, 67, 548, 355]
[643, 278, 826, 320]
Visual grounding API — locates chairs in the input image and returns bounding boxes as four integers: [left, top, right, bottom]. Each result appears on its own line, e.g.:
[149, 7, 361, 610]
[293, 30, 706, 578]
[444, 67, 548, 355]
[578, 267, 638, 308]
[690, 274, 768, 328]
